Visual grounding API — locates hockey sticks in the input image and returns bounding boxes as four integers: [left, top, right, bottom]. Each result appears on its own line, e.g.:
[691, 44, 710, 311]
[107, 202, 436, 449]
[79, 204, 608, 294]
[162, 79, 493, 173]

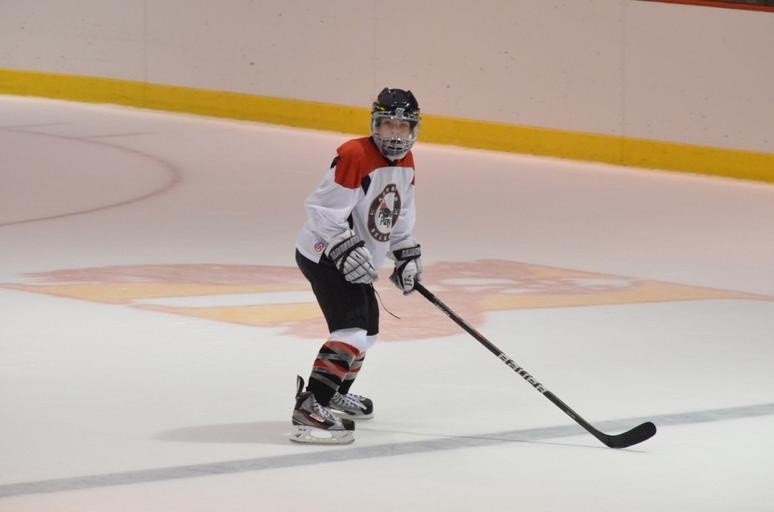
[408, 276, 657, 449]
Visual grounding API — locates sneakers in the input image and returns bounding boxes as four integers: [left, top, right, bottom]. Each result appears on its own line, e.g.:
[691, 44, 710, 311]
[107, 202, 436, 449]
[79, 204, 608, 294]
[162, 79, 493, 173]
[328, 391, 372, 414]
[292, 391, 354, 430]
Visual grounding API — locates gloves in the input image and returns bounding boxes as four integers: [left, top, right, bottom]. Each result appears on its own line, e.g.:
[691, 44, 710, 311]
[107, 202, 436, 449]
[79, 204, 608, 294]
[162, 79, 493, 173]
[389, 244, 423, 295]
[330, 235, 377, 287]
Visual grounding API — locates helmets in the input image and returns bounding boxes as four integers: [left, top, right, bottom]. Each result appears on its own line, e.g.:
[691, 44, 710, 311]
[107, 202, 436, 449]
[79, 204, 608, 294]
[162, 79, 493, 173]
[372, 88, 419, 133]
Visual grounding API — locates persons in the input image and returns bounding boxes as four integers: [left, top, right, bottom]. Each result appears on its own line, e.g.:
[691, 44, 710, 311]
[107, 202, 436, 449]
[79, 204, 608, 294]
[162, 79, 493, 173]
[290, 86, 425, 432]
